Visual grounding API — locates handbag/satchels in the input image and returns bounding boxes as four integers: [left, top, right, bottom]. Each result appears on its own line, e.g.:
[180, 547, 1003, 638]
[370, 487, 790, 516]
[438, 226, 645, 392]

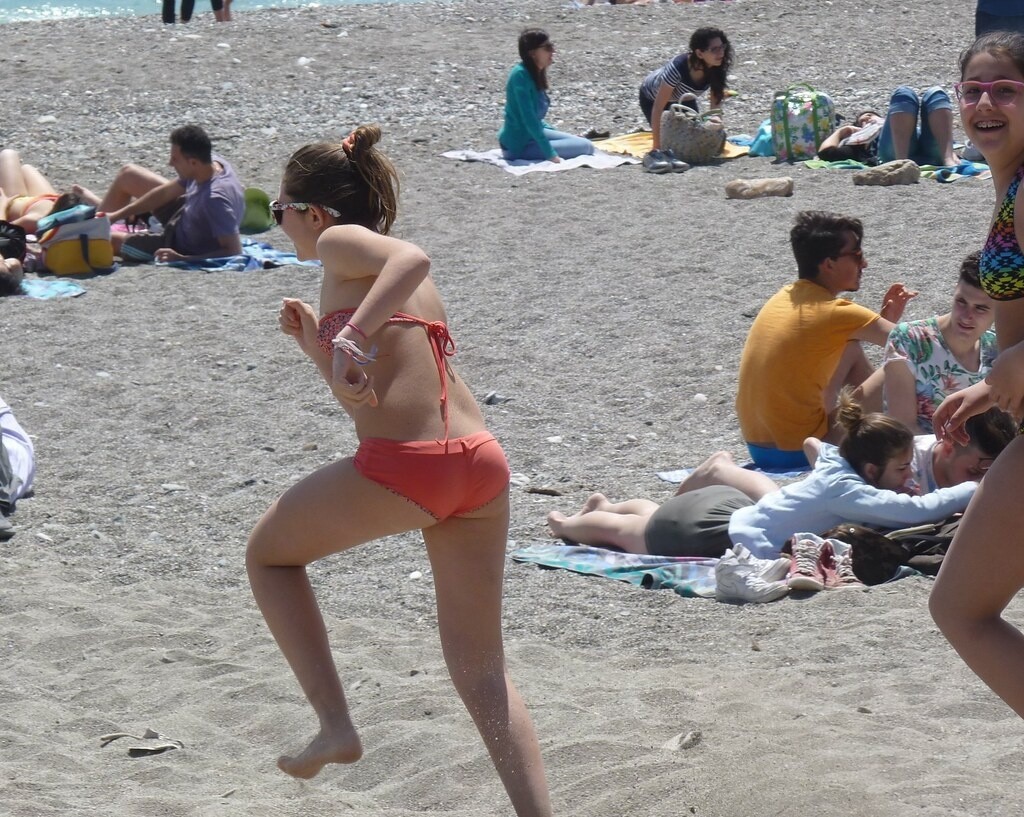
[771, 84, 837, 159]
[40, 215, 118, 276]
[660, 93, 724, 166]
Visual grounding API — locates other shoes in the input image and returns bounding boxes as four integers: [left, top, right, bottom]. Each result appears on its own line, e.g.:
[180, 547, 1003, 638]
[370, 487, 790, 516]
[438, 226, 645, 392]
[628, 125, 653, 133]
[578, 127, 610, 141]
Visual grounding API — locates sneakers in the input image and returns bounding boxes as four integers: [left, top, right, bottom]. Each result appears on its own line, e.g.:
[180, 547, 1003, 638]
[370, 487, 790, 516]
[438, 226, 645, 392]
[642, 149, 673, 174]
[661, 150, 690, 172]
[824, 537, 866, 589]
[716, 549, 789, 602]
[731, 543, 791, 583]
[790, 532, 827, 588]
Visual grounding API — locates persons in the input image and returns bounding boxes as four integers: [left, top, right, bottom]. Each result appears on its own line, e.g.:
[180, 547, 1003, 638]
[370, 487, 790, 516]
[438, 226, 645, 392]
[547, 384, 978, 559]
[72, 125, 555, 817]
[883, 250, 997, 435]
[929, 30, 1024, 718]
[975, 0, 1024, 36]
[817, 84, 961, 167]
[639, 28, 735, 154]
[96, 126, 244, 263]
[737, 211, 918, 474]
[162, 0, 233, 23]
[0, 150, 89, 235]
[498, 28, 595, 164]
[0, 219, 26, 296]
[675, 405, 1019, 503]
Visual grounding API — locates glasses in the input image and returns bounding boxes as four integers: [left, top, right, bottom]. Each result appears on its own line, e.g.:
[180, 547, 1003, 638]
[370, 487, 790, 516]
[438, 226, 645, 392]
[269, 200, 344, 225]
[536, 44, 555, 52]
[830, 243, 863, 263]
[954, 79, 1024, 107]
[965, 444, 997, 470]
[703, 43, 727, 54]
